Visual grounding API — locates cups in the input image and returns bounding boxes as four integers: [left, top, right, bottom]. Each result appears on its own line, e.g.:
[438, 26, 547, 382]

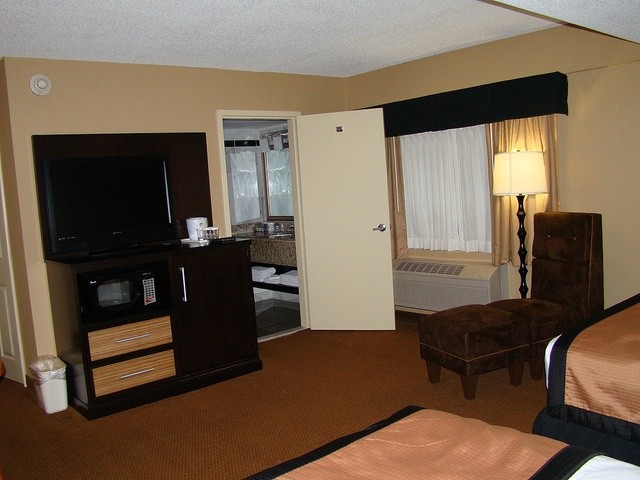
[205, 227, 219, 239]
[186, 217, 208, 240]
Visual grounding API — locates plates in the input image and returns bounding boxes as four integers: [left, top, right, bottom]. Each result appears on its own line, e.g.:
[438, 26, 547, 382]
[180, 239, 209, 243]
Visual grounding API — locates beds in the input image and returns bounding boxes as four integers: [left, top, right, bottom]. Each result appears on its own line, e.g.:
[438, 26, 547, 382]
[532, 290, 640, 469]
[242, 404, 640, 479]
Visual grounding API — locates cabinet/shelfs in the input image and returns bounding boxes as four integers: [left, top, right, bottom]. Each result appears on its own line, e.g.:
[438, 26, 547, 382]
[42, 236, 263, 421]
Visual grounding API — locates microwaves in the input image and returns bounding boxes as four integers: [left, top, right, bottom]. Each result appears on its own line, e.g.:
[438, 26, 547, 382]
[75, 259, 173, 325]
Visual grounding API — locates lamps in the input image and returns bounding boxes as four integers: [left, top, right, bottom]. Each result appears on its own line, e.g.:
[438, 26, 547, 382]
[492, 152, 548, 301]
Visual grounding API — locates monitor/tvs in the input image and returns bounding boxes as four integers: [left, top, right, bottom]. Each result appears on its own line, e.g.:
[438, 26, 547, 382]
[31, 133, 214, 266]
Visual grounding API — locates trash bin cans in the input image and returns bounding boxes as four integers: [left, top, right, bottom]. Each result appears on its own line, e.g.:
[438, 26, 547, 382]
[30, 357, 70, 416]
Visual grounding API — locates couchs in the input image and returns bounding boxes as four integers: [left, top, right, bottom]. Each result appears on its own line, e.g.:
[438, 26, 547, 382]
[418, 213, 603, 399]
